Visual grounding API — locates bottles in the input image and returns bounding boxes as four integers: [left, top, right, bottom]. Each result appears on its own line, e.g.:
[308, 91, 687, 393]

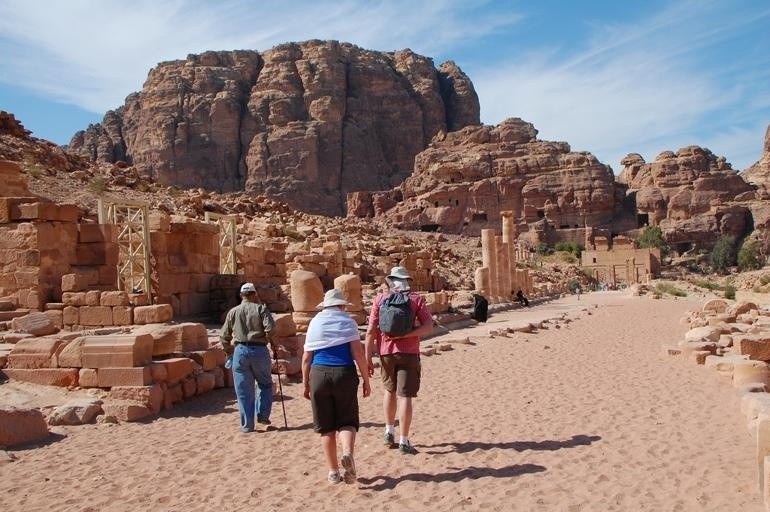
[224, 355, 233, 369]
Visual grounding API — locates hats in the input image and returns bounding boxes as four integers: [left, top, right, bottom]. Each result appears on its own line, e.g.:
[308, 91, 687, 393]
[384, 266, 414, 282]
[315, 288, 353, 311]
[240, 282, 257, 294]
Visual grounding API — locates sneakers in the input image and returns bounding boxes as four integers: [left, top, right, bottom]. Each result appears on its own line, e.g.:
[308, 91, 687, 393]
[340, 451, 357, 484]
[383, 432, 395, 448]
[397, 439, 419, 456]
[256, 418, 271, 425]
[327, 470, 342, 485]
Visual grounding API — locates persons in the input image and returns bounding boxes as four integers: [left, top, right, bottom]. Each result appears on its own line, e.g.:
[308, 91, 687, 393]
[576, 280, 616, 301]
[511, 290, 519, 302]
[220, 282, 277, 435]
[301, 289, 371, 486]
[363, 266, 435, 457]
[518, 289, 531, 307]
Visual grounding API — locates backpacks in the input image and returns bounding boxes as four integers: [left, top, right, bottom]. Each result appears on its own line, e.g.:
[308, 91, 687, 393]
[379, 291, 414, 338]
